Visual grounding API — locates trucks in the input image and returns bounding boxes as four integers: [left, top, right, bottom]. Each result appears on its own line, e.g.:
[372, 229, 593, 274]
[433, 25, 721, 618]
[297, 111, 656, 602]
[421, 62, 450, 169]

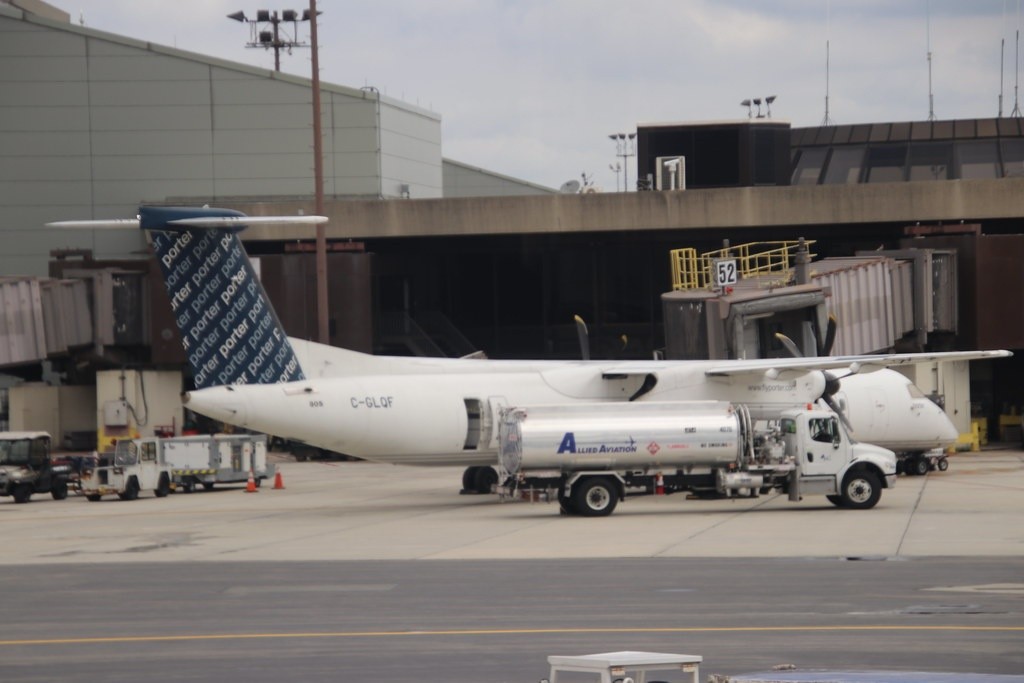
[81, 436, 173, 501]
[497, 400, 896, 514]
[0, 431, 69, 502]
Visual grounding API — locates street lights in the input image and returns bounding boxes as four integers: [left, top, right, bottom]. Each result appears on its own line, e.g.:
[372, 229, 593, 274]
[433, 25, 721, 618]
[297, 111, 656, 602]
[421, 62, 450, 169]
[227, 8, 322, 71]
[611, 133, 635, 191]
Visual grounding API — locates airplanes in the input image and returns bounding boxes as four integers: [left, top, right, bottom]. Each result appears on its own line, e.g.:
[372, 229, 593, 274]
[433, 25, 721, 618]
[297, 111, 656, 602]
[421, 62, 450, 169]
[44, 203, 1015, 495]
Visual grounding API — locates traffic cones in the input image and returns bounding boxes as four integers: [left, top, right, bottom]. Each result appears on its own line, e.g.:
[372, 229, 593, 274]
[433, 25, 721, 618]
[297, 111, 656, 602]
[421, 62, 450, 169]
[246, 468, 256, 492]
[274, 465, 285, 490]
